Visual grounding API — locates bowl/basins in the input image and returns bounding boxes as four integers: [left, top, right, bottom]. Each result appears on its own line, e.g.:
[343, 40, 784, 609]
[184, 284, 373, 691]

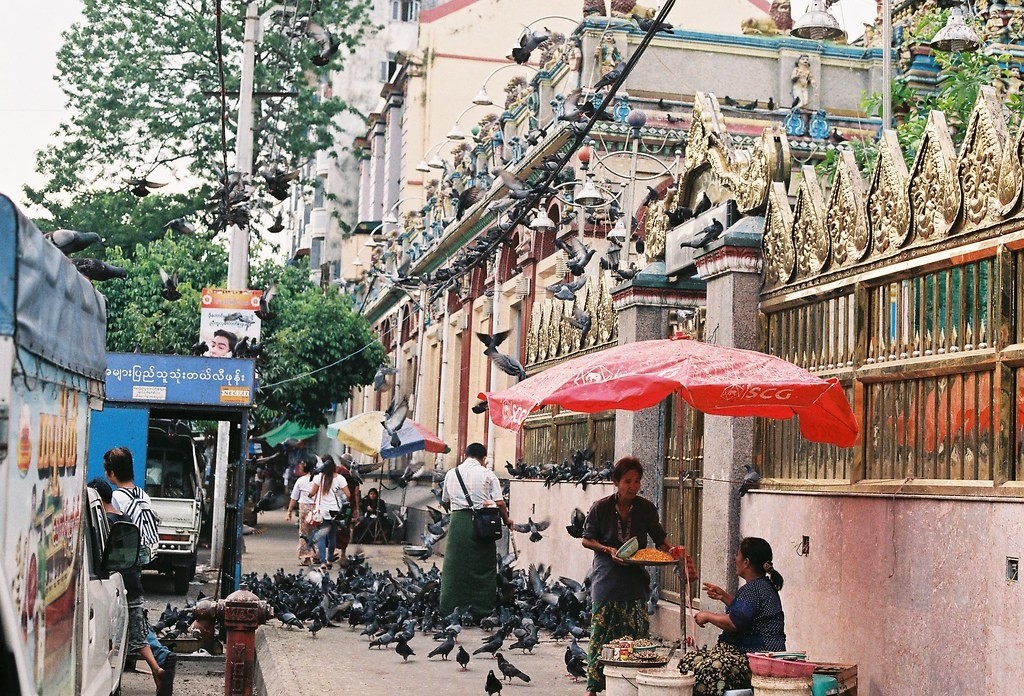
[622, 640, 657, 653]
[403, 546, 429, 556]
[155, 637, 206, 654]
[615, 536, 639, 558]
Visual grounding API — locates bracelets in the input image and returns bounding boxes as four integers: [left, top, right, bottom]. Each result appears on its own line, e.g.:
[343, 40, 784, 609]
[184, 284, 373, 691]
[668, 547, 675, 554]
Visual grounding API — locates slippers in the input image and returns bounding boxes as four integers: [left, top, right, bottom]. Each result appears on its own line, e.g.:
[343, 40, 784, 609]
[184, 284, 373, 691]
[297, 562, 309, 566]
[157, 670, 172, 696]
[313, 560, 321, 564]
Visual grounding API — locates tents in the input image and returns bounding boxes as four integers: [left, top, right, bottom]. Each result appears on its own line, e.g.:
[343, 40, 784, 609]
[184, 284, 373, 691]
[88, 355, 256, 597]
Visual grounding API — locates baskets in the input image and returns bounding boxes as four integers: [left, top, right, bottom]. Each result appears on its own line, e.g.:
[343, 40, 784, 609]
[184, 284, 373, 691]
[746, 653, 818, 679]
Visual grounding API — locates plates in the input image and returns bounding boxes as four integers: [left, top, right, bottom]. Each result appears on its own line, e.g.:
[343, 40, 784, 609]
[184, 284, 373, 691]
[597, 657, 670, 668]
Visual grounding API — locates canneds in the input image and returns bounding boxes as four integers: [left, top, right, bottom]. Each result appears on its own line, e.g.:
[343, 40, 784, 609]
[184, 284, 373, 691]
[619, 648, 628, 661]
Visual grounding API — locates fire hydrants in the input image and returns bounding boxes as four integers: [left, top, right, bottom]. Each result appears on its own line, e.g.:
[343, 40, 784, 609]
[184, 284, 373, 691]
[191, 584, 275, 696]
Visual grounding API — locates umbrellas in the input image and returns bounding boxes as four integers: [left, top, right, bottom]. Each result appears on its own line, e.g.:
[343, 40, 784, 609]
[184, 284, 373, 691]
[476, 331, 862, 660]
[327, 411, 450, 542]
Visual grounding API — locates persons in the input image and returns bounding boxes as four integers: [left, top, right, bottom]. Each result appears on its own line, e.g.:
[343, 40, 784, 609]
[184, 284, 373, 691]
[790, 55, 816, 109]
[439, 443, 514, 625]
[250, 444, 393, 574]
[87, 447, 177, 696]
[353, 35, 582, 310]
[677, 538, 786, 696]
[582, 458, 676, 696]
[208, 329, 237, 359]
[593, 30, 624, 91]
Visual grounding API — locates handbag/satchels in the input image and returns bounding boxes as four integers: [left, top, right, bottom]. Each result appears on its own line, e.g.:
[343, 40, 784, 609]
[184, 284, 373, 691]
[472, 511, 502, 540]
[304, 511, 323, 527]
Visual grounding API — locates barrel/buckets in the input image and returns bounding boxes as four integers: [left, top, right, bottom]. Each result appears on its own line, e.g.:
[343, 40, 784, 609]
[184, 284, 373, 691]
[603, 665, 668, 695]
[751, 674, 813, 696]
[636, 668, 696, 696]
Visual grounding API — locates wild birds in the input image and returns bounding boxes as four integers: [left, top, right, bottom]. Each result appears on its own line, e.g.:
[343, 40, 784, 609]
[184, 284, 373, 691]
[157, 263, 184, 302]
[68, 259, 128, 283]
[655, 94, 852, 148]
[41, 227, 107, 256]
[154, 447, 660, 696]
[298, 19, 342, 70]
[474, 329, 514, 354]
[188, 335, 269, 362]
[380, 394, 410, 446]
[489, 354, 529, 383]
[418, 58, 629, 338]
[162, 169, 302, 234]
[644, 184, 724, 250]
[735, 461, 763, 500]
[121, 174, 168, 197]
[511, 33, 550, 64]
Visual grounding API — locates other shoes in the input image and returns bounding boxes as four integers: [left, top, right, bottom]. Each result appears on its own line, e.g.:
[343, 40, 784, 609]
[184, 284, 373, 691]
[160, 652, 177, 681]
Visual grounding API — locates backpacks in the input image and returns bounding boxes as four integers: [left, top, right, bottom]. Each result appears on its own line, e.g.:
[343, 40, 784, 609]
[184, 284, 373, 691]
[115, 486, 159, 566]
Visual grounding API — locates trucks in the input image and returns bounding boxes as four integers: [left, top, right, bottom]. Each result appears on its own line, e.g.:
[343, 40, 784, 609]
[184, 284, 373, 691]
[149, 419, 212, 596]
[0, 193, 142, 696]
